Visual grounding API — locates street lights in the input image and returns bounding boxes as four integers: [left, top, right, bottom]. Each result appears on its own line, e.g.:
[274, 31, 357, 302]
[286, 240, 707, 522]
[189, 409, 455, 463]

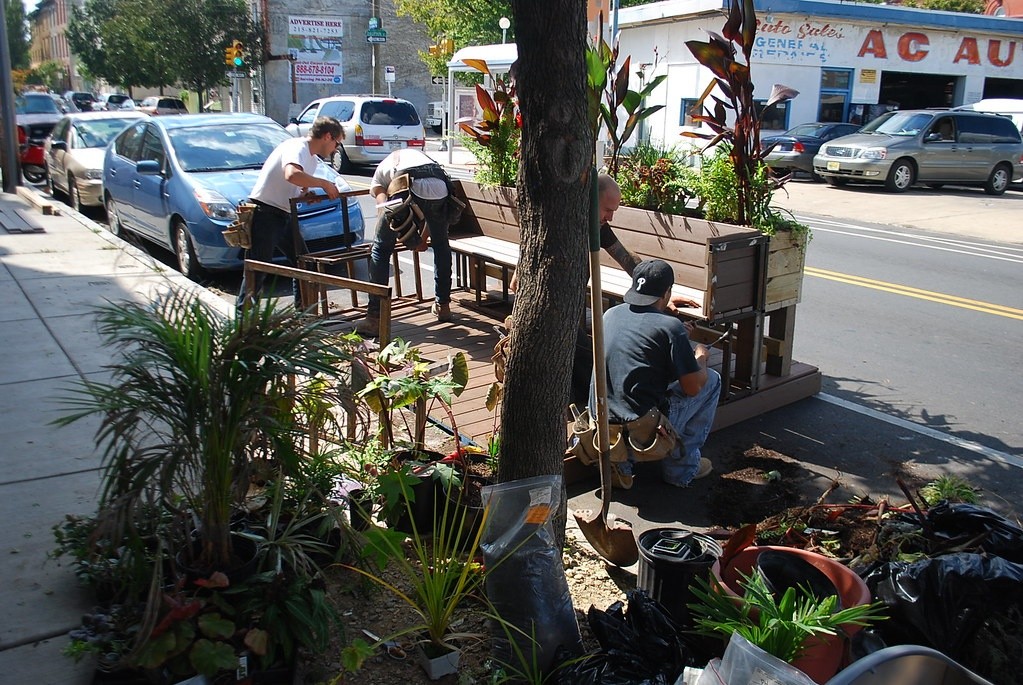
[499, 15, 511, 46]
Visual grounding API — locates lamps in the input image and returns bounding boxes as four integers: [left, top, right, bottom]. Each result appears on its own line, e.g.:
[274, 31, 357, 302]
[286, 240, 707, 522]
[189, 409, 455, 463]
[765, 11, 773, 23]
[635, 71, 644, 78]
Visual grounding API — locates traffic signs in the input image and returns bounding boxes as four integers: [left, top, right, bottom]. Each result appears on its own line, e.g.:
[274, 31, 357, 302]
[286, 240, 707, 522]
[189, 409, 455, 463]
[432, 77, 455, 85]
[365, 36, 386, 44]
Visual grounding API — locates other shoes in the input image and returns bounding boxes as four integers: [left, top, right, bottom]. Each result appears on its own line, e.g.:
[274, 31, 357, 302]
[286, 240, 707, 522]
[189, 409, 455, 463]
[432, 303, 450, 320]
[611, 462, 633, 489]
[352, 314, 380, 336]
[662, 457, 712, 485]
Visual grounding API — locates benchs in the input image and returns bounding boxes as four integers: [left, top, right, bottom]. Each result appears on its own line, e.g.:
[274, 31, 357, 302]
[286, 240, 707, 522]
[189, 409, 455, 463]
[289, 179, 760, 406]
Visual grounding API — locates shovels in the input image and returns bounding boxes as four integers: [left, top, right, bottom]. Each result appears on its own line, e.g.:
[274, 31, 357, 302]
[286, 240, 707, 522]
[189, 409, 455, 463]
[572, 165, 639, 567]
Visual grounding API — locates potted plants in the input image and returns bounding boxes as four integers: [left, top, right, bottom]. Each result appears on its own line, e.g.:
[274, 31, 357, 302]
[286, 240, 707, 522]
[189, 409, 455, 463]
[709, 544, 872, 685]
[420, 350, 504, 550]
[325, 462, 565, 685]
[341, 330, 465, 534]
[440, 0, 813, 313]
[911, 471, 985, 511]
[41, 244, 353, 685]
[333, 423, 398, 533]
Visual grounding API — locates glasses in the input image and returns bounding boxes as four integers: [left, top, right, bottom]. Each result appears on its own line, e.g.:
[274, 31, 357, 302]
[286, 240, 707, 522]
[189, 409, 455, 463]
[331, 134, 341, 148]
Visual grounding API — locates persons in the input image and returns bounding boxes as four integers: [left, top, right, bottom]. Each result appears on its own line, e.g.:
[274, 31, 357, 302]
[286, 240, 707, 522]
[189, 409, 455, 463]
[237, 117, 345, 320]
[589, 260, 722, 488]
[510, 175, 702, 400]
[351, 148, 454, 335]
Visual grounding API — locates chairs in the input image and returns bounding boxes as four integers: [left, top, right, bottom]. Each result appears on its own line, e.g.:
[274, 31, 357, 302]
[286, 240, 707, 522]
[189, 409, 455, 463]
[939, 122, 953, 140]
[77, 131, 102, 147]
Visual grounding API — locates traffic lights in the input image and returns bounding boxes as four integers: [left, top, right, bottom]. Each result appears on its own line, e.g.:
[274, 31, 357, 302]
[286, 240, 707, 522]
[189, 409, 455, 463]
[226, 48, 234, 64]
[429, 39, 454, 56]
[232, 40, 244, 67]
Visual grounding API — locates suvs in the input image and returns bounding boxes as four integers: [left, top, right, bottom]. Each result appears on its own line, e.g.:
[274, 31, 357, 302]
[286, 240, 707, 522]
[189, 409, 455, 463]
[284, 94, 426, 172]
[426, 99, 448, 135]
[760, 99, 1023, 197]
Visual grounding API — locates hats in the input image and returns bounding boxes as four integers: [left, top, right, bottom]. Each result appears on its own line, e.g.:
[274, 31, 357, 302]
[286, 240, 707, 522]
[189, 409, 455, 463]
[623, 259, 674, 306]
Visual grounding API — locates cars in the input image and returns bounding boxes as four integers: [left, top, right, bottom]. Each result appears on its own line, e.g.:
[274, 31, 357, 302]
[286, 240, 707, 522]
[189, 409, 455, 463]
[103, 112, 367, 281]
[13, 85, 189, 214]
